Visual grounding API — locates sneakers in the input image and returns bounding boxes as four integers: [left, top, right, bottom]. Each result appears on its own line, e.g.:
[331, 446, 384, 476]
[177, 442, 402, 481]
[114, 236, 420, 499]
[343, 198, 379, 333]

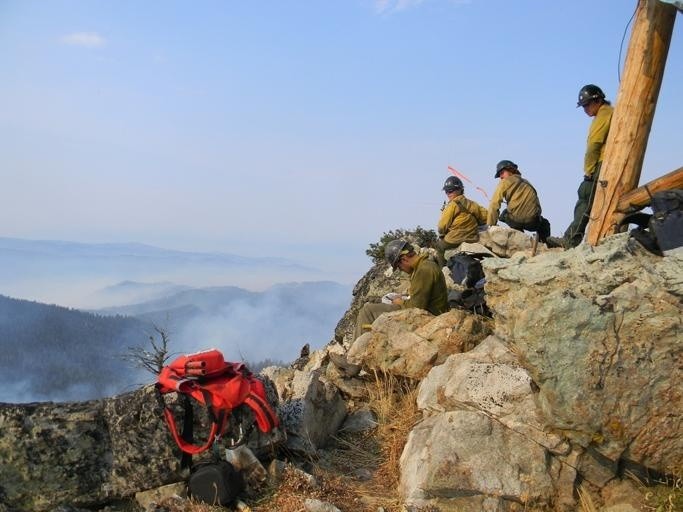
[328, 352, 362, 376]
[545, 236, 565, 247]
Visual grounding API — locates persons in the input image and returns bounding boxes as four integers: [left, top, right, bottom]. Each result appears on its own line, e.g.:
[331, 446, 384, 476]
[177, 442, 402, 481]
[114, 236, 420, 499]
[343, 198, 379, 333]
[329, 240, 448, 376]
[484, 160, 541, 232]
[434, 175, 486, 264]
[545, 83, 616, 245]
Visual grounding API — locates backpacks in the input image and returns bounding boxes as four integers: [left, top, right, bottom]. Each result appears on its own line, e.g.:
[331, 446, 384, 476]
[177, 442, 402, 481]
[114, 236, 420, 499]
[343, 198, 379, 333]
[614, 189, 682, 257]
[154, 348, 278, 454]
[446, 251, 492, 319]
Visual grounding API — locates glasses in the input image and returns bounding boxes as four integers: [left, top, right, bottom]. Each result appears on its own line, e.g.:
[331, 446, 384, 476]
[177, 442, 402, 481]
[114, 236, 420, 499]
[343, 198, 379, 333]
[445, 189, 455, 193]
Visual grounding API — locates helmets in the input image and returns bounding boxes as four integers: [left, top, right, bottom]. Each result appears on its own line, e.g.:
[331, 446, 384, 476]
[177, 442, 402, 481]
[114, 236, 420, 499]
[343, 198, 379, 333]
[494, 160, 514, 178]
[443, 176, 463, 190]
[385, 240, 414, 273]
[575, 84, 605, 108]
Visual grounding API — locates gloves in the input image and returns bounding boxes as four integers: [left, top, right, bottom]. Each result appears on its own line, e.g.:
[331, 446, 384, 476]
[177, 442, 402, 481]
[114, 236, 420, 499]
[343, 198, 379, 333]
[225, 441, 269, 494]
[233, 415, 286, 450]
